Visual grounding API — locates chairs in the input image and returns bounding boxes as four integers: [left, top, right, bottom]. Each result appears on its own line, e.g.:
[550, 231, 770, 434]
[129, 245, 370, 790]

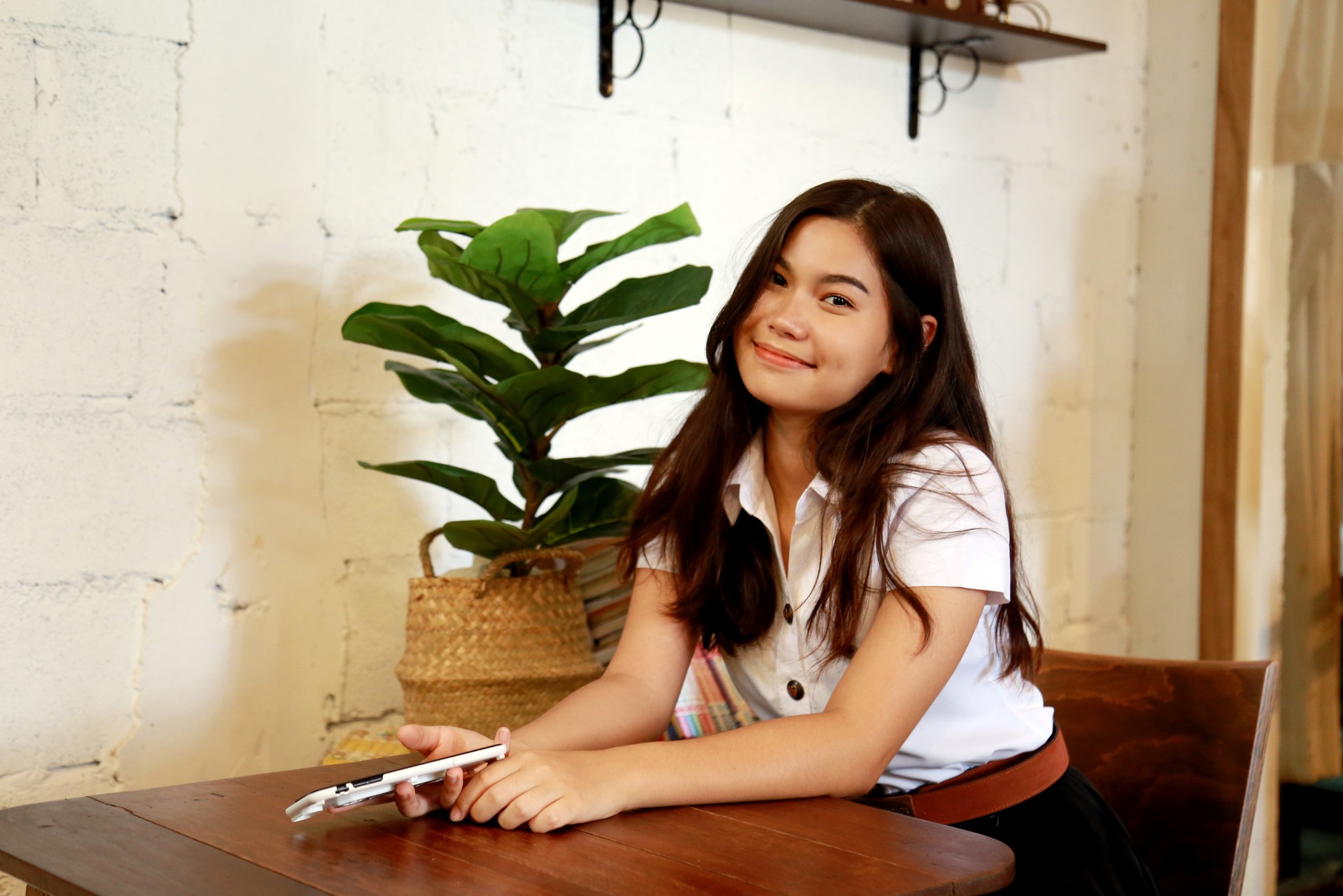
[1015, 641, 1283, 896]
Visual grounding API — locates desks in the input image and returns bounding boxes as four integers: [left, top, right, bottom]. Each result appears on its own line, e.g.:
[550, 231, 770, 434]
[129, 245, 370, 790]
[0, 745, 1015, 896]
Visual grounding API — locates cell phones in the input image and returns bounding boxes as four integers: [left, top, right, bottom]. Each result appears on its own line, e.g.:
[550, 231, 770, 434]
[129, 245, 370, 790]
[287, 743, 506, 822]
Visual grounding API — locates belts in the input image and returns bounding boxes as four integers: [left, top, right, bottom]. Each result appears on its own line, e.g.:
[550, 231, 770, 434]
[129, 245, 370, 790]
[856, 723, 1071, 824]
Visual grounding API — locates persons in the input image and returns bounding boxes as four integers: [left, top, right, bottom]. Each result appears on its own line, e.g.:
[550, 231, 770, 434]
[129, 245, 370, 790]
[331, 180, 1130, 896]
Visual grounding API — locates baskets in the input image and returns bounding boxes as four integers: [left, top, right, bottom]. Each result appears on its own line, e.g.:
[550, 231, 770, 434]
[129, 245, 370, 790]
[396, 526, 603, 739]
